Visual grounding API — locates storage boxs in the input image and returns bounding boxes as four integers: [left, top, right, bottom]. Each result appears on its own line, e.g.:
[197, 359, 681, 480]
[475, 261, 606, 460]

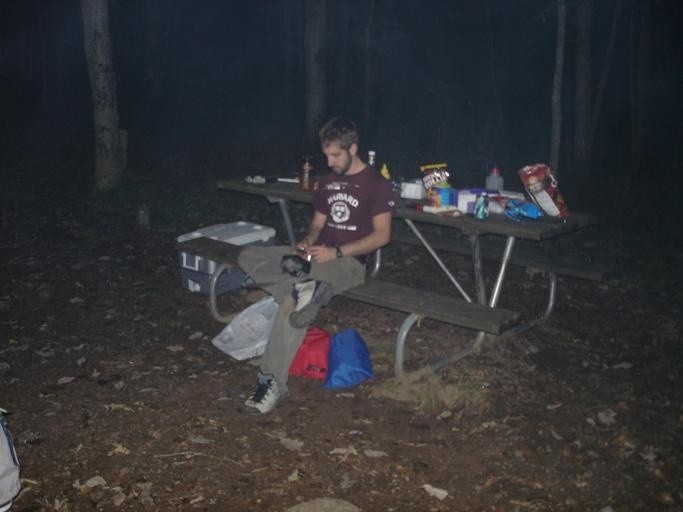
[176, 221, 277, 294]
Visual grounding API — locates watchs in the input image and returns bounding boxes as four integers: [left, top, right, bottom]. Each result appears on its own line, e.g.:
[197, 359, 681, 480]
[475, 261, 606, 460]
[336, 246, 343, 258]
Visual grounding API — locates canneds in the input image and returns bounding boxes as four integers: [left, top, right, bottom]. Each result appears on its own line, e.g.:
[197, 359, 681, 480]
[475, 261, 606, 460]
[473, 192, 489, 221]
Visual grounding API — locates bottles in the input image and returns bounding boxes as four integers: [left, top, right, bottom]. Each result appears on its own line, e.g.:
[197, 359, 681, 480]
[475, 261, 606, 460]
[367, 151, 378, 168]
[380, 163, 389, 179]
[486, 167, 504, 191]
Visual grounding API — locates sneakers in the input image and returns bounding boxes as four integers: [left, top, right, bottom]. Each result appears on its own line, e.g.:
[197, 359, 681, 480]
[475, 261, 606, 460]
[289, 278, 333, 329]
[237, 371, 291, 416]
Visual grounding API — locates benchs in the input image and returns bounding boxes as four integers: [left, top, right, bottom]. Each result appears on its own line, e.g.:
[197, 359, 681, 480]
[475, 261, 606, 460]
[372, 221, 616, 319]
[174, 236, 522, 381]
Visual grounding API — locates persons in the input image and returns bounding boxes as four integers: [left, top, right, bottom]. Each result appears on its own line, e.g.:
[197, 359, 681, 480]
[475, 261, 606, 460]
[240, 116, 397, 416]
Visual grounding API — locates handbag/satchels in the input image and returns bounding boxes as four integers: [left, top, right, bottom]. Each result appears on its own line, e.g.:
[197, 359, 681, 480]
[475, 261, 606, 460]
[210, 295, 373, 389]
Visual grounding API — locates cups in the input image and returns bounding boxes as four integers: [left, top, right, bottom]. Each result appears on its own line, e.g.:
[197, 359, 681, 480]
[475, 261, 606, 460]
[431, 181, 452, 207]
[297, 152, 314, 193]
